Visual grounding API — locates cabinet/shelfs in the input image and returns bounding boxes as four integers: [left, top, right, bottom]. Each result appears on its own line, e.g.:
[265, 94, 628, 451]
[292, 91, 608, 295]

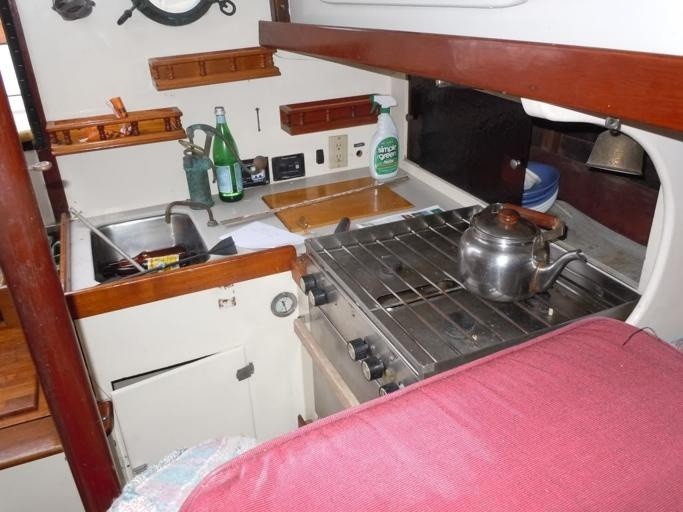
[43, 104, 187, 157]
[144, 46, 283, 93]
[279, 93, 383, 136]
[111, 345, 257, 480]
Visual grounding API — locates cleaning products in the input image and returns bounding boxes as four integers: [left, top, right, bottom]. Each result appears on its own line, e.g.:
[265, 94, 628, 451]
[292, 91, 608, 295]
[368, 95, 401, 180]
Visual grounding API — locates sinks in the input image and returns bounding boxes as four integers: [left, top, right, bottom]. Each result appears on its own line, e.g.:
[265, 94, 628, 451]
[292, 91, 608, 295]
[89, 211, 211, 284]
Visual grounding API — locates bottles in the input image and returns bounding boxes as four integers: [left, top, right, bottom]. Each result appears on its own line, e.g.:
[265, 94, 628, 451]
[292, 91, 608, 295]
[210, 107, 242, 203]
[102, 245, 187, 280]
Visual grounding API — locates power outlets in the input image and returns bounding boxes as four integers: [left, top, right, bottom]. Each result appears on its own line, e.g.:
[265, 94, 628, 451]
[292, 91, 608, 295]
[328, 135, 349, 169]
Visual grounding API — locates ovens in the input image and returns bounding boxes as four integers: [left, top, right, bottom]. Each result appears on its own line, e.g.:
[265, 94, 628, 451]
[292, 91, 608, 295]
[293, 255, 422, 420]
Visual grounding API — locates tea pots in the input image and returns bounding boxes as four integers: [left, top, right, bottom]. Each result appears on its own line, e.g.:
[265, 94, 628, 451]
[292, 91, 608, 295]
[457, 200, 588, 304]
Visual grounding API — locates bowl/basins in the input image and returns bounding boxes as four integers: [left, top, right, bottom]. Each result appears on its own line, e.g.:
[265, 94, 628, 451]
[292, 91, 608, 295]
[521, 161, 562, 214]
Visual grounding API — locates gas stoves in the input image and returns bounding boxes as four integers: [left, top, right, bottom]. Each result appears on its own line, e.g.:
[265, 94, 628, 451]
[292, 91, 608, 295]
[304, 203, 640, 378]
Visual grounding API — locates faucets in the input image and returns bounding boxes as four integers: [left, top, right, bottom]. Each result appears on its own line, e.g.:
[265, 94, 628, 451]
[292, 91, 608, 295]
[163, 201, 217, 227]
